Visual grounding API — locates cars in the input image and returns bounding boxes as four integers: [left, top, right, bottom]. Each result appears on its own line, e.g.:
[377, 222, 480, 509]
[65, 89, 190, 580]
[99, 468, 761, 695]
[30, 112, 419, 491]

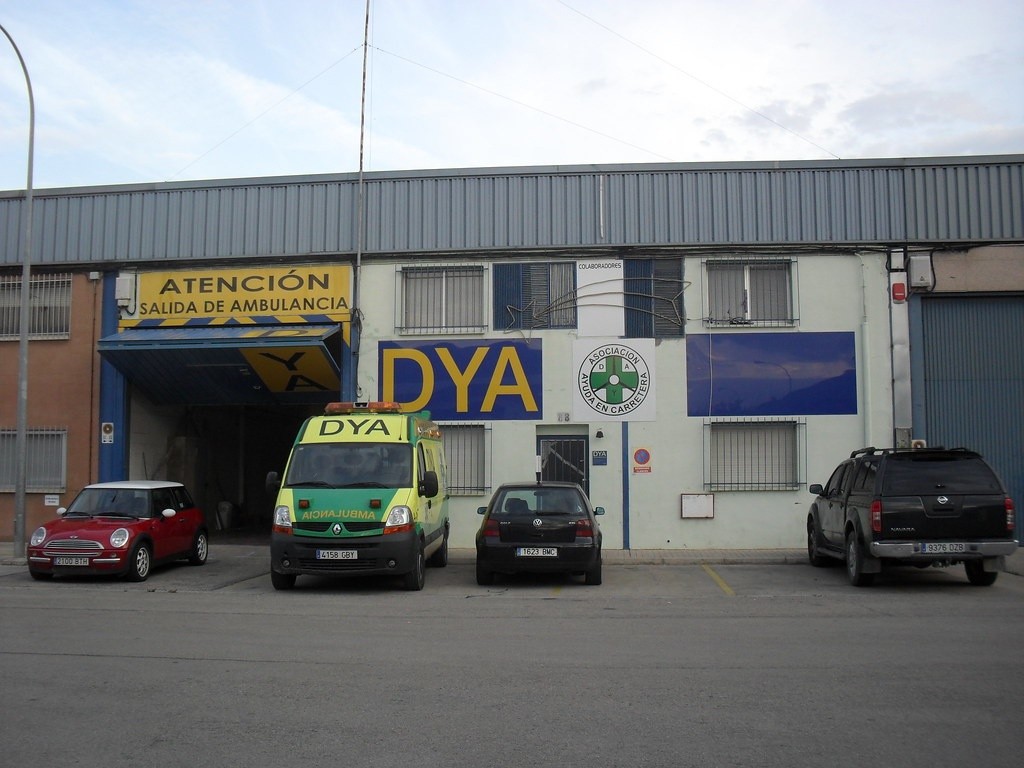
[26, 480, 210, 582]
[476, 481, 605, 586]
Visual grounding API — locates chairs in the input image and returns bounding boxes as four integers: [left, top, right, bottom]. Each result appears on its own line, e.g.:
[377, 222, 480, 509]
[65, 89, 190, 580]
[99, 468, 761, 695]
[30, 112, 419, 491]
[310, 453, 411, 482]
[505, 498, 528, 512]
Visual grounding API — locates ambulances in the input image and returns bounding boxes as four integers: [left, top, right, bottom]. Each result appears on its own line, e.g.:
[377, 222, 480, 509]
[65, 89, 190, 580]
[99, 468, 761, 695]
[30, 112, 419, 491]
[269, 402, 452, 592]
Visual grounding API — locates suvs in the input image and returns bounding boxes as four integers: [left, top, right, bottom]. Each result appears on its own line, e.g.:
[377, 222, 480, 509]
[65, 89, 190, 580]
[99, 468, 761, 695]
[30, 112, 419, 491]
[806, 445, 1018, 587]
